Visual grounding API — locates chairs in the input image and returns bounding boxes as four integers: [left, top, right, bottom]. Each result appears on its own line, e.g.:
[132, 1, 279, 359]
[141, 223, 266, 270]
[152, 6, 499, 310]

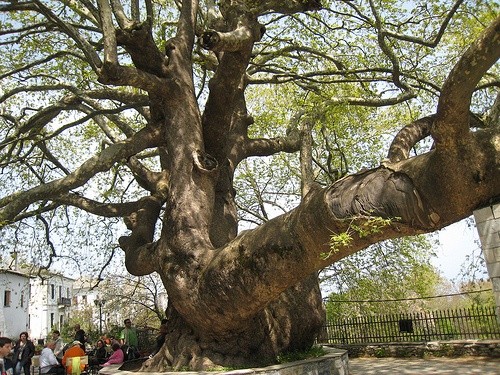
[65, 355, 89, 375]
[31, 355, 40, 375]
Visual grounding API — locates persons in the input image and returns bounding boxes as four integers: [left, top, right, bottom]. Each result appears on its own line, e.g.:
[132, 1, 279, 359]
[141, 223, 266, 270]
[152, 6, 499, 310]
[38, 342, 65, 375]
[87, 339, 107, 370]
[98, 343, 124, 370]
[62, 340, 86, 375]
[110, 336, 121, 349]
[143, 319, 174, 362]
[68, 324, 88, 353]
[120, 319, 139, 361]
[48, 330, 64, 365]
[13, 331, 35, 375]
[0, 337, 13, 375]
[104, 337, 113, 358]
